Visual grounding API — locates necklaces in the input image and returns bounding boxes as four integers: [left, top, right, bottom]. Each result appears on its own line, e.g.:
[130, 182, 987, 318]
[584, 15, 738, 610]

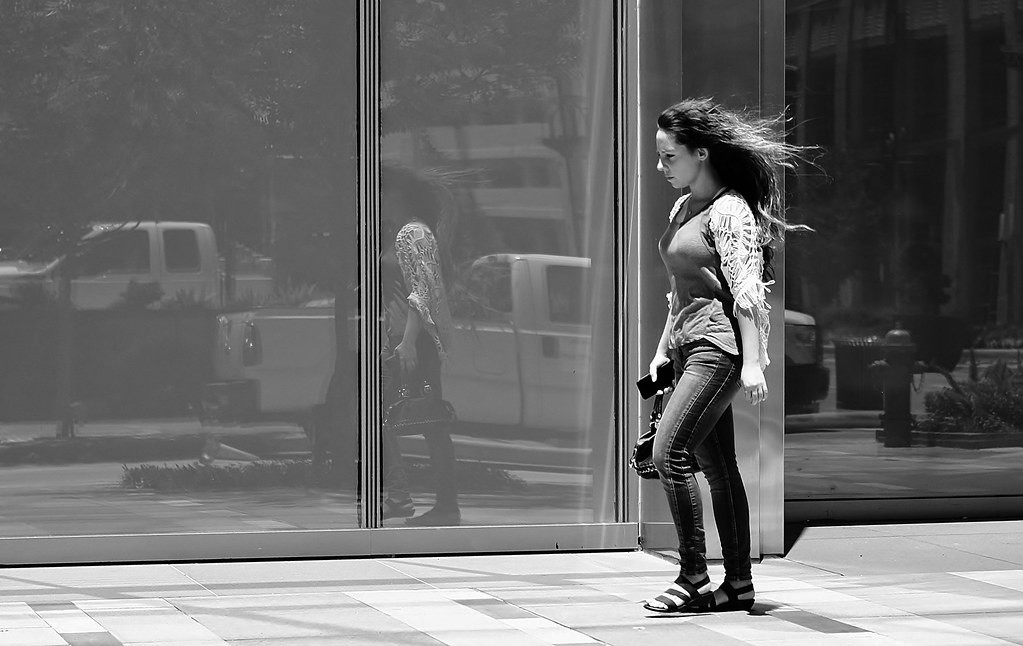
[688, 196, 714, 215]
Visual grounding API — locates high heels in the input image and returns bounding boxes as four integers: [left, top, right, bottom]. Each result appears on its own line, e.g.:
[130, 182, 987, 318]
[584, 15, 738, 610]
[406, 507, 462, 528]
[689, 580, 754, 611]
[644, 573, 712, 613]
[382, 496, 416, 520]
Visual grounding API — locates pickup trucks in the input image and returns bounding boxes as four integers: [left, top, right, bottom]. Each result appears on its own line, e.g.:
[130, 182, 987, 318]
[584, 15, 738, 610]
[207, 250, 818, 433]
[0, 217, 278, 315]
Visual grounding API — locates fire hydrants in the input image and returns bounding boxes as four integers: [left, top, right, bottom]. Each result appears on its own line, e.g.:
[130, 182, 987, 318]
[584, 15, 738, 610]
[866, 325, 924, 449]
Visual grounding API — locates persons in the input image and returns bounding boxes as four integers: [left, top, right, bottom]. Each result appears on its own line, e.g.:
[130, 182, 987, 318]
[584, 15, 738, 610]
[643, 95, 780, 614]
[382, 158, 505, 528]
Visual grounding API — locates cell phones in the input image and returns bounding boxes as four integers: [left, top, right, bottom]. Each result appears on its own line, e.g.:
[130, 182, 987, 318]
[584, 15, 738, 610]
[384, 355, 402, 375]
[636, 360, 676, 401]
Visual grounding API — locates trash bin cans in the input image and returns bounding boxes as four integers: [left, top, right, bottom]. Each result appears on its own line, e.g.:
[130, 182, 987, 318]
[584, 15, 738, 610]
[830, 336, 885, 412]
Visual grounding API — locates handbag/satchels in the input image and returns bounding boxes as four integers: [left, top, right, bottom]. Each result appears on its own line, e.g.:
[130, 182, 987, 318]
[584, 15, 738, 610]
[629, 386, 701, 479]
[382, 360, 457, 436]
